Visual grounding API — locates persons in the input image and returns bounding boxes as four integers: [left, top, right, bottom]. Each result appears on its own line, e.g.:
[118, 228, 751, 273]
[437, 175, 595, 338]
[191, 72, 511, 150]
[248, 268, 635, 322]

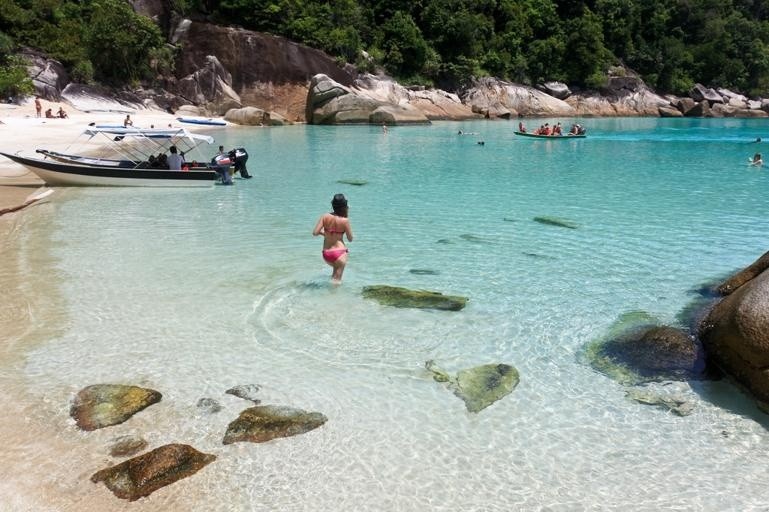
[56, 107, 69, 119]
[749, 154, 762, 167]
[216, 145, 225, 154]
[517, 121, 588, 139]
[167, 146, 185, 171]
[46, 109, 59, 119]
[312, 193, 353, 283]
[124, 115, 133, 128]
[35, 96, 41, 117]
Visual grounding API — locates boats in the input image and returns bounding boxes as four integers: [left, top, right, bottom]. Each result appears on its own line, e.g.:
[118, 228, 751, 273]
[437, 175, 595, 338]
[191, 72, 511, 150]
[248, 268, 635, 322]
[0, 125, 218, 188]
[512, 130, 587, 140]
[175, 116, 228, 127]
[34, 120, 238, 179]
